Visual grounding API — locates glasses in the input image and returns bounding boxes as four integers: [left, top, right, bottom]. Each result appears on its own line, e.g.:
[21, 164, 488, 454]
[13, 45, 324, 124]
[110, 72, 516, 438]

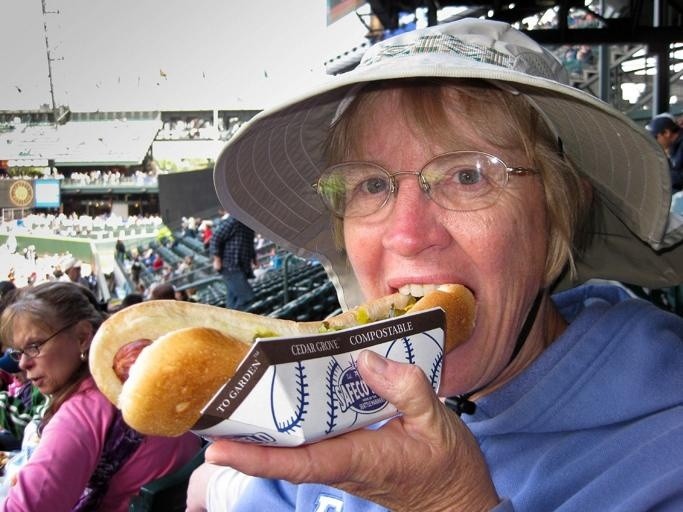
[311, 150, 538, 218]
[9, 317, 82, 361]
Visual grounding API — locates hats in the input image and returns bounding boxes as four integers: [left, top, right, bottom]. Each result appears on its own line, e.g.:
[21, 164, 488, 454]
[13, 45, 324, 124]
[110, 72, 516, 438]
[213, 17, 682, 313]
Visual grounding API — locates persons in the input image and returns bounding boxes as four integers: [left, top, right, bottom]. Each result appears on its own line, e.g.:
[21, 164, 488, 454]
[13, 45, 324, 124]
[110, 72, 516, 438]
[643, 111, 682, 187]
[1, 284, 203, 511]
[185, 17, 682, 511]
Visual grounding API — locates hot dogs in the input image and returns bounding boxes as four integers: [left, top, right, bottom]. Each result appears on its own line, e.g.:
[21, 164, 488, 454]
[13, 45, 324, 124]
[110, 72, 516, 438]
[86, 283, 477, 437]
